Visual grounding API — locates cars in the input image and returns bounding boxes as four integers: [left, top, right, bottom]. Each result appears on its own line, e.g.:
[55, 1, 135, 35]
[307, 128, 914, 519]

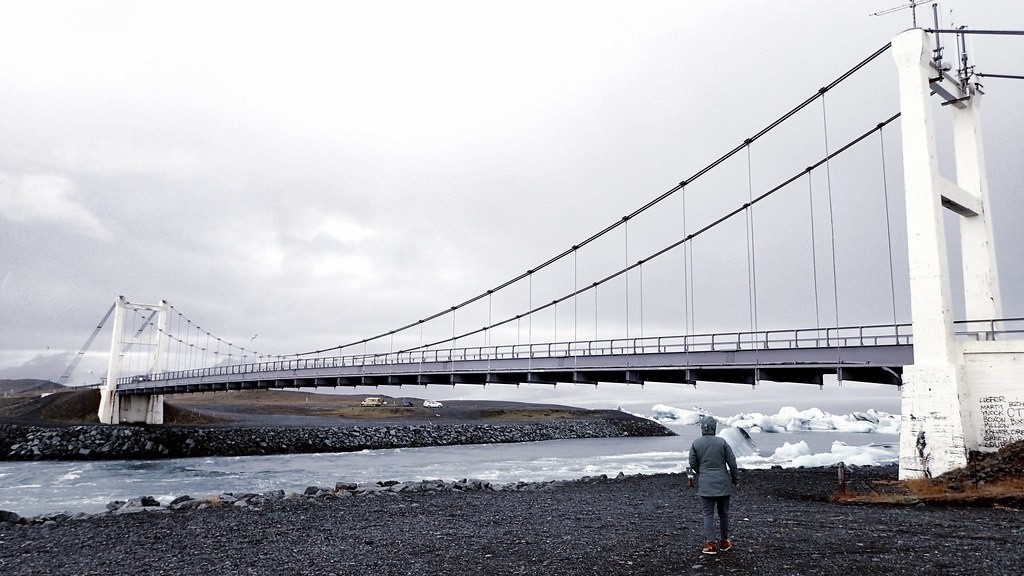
[403, 401, 413, 407]
[423, 400, 443, 408]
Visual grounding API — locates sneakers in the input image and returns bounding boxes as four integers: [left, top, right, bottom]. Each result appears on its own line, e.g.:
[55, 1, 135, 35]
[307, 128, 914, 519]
[702, 540, 717, 554]
[719, 539, 732, 551]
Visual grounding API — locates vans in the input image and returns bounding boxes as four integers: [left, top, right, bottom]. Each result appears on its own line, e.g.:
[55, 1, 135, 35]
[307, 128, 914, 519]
[360, 397, 383, 407]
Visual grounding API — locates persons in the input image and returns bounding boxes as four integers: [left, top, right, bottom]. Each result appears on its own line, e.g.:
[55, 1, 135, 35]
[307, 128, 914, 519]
[688, 417, 739, 554]
